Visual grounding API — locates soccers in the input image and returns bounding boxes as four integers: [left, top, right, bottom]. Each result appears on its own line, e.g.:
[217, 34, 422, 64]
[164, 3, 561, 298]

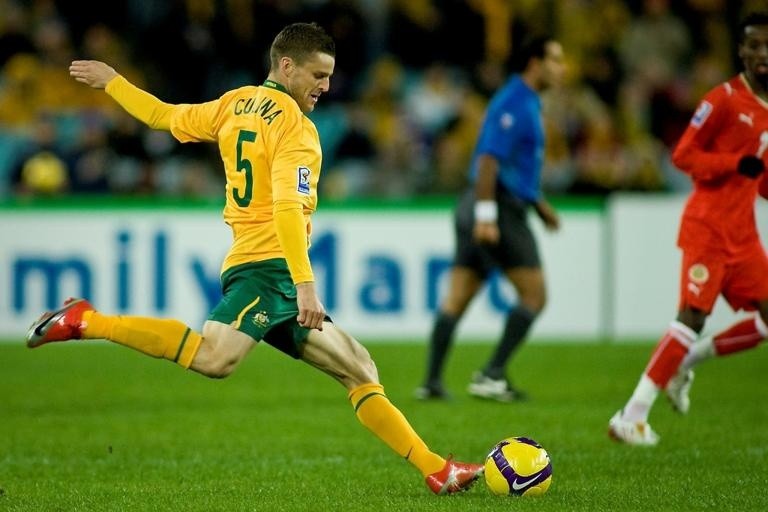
[484, 437, 552, 497]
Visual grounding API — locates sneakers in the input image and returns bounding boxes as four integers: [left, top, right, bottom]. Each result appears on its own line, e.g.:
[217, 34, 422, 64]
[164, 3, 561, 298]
[666, 370, 695, 414]
[26, 297, 95, 348]
[470, 376, 519, 402]
[426, 453, 485, 495]
[607, 410, 660, 447]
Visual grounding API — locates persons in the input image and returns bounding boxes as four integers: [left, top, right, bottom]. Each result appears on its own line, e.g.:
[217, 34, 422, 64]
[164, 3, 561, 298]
[1, 0, 766, 192]
[416, 35, 559, 401]
[606, 12, 767, 449]
[25, 22, 486, 495]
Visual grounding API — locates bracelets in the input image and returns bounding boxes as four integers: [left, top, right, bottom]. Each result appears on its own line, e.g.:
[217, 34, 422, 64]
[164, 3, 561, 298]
[475, 200, 500, 224]
